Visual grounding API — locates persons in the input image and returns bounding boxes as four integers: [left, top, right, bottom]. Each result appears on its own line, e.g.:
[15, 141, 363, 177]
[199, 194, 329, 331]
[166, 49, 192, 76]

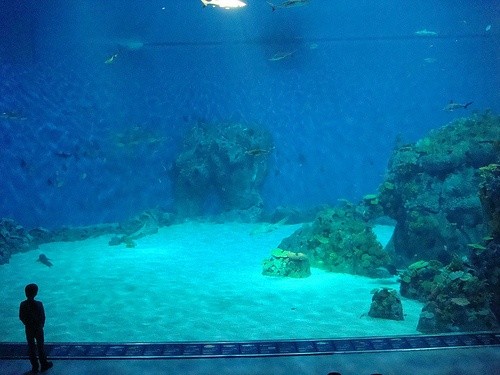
[19, 283, 53, 372]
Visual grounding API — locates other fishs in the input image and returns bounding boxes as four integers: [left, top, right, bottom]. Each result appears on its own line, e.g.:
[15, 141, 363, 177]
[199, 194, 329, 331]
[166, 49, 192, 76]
[250, 215, 291, 235]
[35, 253, 54, 267]
[264, 0, 306, 12]
[201, 0, 247, 9]
[124, 223, 146, 239]
[245, 149, 271, 156]
[267, 49, 298, 60]
[443, 99, 473, 111]
[104, 52, 120, 64]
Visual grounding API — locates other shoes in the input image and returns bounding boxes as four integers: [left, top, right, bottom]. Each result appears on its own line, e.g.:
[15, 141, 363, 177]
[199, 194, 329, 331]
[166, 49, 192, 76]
[41, 362, 53, 371]
